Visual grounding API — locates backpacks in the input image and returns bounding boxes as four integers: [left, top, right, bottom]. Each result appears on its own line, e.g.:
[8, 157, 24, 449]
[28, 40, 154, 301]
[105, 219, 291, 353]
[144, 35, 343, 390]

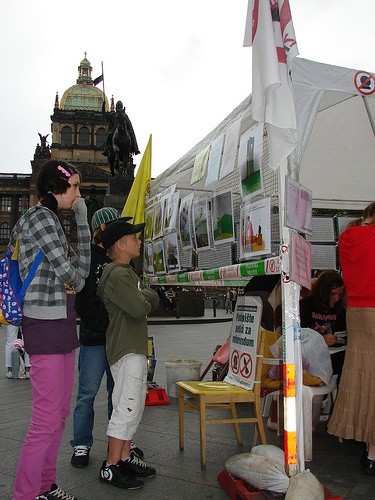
[0, 237, 44, 326]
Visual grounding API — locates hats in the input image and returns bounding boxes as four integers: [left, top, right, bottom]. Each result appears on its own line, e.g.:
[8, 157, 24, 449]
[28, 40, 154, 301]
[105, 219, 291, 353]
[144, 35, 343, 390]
[101, 222, 146, 250]
[92, 207, 133, 231]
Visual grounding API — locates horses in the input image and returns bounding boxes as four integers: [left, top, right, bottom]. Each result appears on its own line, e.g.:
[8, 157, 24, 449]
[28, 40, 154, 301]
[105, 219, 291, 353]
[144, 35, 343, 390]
[105, 106, 132, 180]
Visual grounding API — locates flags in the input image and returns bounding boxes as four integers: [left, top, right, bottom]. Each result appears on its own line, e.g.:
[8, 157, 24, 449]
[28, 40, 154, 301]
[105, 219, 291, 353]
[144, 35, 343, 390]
[243, 0, 301, 173]
[121, 134, 152, 239]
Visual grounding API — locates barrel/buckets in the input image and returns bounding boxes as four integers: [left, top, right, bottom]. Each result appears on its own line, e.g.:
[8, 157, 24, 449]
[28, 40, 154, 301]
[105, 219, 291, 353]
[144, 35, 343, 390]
[164, 359, 203, 397]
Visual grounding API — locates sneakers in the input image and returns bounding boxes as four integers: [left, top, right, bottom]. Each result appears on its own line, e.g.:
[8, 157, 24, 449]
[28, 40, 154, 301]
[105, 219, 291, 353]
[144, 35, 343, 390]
[107, 439, 143, 457]
[71, 445, 90, 468]
[116, 452, 156, 478]
[35, 494, 55, 500]
[99, 460, 144, 490]
[47, 483, 77, 500]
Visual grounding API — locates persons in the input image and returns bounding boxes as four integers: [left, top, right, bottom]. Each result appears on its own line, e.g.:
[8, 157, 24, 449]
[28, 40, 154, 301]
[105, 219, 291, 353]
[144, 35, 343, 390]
[328, 203, 375, 475]
[101, 100, 141, 155]
[224, 287, 237, 314]
[155, 286, 202, 311]
[69, 208, 144, 468]
[99, 220, 160, 490]
[10, 160, 91, 500]
[5, 325, 32, 380]
[299, 270, 347, 398]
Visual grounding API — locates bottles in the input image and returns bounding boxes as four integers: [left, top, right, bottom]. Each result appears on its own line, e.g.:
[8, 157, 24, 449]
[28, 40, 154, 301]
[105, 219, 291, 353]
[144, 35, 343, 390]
[11, 348, 20, 379]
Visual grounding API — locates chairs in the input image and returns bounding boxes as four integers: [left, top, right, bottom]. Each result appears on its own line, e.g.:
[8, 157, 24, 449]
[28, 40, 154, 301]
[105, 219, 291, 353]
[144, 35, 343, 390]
[252, 327, 343, 462]
[176, 324, 267, 471]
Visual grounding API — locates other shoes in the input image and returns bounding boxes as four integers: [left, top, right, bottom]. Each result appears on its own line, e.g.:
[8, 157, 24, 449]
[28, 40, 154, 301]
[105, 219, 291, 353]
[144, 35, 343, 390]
[360, 452, 368, 465]
[362, 458, 375, 475]
[6, 371, 13, 379]
[25, 372, 30, 379]
